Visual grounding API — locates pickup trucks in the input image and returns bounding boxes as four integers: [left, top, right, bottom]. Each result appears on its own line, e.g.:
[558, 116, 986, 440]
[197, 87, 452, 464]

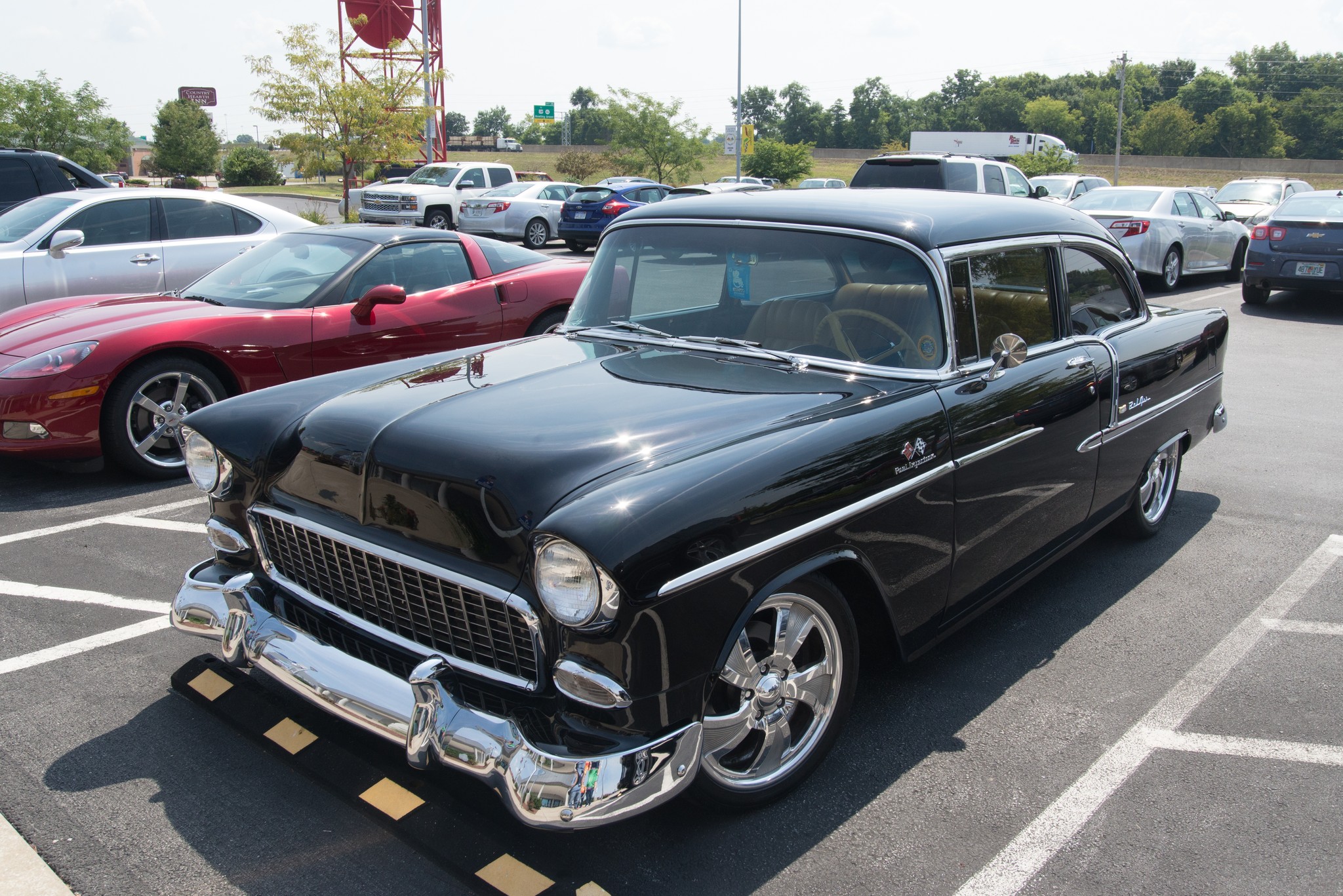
[358, 161, 575, 231]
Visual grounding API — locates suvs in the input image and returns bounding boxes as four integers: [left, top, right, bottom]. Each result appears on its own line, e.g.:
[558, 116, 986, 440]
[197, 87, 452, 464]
[1201, 177, 1318, 234]
[851, 151, 1047, 201]
[1026, 173, 1111, 209]
[1174, 185, 1219, 215]
[0, 145, 129, 210]
[338, 159, 555, 216]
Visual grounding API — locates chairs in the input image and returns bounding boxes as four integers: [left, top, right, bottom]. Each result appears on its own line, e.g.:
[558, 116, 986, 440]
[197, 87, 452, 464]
[355, 248, 396, 303]
[402, 250, 456, 294]
[742, 294, 836, 357]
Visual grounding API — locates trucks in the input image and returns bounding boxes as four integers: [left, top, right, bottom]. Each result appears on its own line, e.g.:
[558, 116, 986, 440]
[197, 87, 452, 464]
[214, 156, 241, 181]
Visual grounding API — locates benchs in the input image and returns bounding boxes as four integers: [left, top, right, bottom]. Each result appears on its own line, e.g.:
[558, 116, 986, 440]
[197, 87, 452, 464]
[837, 280, 1053, 354]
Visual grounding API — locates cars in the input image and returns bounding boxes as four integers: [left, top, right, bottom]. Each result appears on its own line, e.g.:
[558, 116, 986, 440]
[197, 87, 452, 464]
[557, 176, 847, 262]
[1243, 190, 1343, 307]
[170, 188, 1229, 831]
[175, 172, 188, 181]
[458, 182, 604, 249]
[148, 169, 159, 177]
[1064, 187, 1250, 292]
[1, 188, 354, 313]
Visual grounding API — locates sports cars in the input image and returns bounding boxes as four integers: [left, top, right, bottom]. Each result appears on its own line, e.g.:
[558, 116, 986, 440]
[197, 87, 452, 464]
[0, 224, 629, 484]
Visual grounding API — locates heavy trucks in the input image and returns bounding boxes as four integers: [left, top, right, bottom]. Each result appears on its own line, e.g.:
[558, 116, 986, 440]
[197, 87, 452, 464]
[910, 132, 1078, 173]
[446, 136, 523, 153]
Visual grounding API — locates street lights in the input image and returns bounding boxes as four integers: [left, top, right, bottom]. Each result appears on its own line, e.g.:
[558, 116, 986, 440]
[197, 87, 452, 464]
[224, 113, 228, 143]
[253, 125, 259, 149]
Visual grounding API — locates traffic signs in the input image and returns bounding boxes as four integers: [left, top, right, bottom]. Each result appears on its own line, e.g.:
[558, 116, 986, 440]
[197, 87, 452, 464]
[534, 105, 555, 124]
